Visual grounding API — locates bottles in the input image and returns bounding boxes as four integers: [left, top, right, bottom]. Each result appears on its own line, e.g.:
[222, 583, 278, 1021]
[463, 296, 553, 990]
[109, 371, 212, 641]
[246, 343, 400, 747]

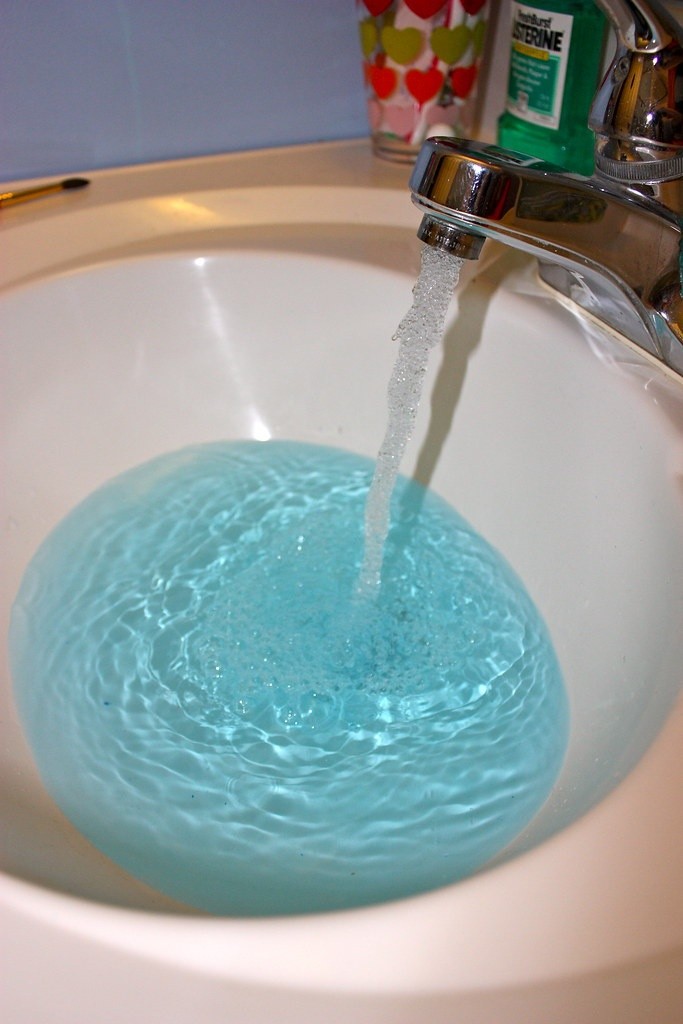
[492, 0, 609, 177]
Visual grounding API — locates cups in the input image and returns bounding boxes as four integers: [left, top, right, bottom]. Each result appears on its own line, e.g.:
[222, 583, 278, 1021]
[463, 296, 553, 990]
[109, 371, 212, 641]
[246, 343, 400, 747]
[356, 0, 497, 166]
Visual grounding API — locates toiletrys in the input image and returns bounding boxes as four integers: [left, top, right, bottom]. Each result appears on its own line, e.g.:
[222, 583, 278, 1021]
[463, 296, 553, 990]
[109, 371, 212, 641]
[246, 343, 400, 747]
[493, 0, 612, 180]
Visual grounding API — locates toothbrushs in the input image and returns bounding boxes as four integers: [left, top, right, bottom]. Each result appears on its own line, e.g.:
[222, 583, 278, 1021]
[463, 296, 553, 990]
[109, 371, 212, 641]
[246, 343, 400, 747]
[408, 0, 473, 145]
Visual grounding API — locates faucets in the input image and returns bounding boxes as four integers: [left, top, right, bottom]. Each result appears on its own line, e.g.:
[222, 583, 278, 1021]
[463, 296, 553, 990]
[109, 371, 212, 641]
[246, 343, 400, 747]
[402, 0, 681, 382]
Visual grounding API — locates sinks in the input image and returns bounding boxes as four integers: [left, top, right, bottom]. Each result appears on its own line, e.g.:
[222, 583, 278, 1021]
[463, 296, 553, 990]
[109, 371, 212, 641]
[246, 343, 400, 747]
[0, 126, 683, 1024]
